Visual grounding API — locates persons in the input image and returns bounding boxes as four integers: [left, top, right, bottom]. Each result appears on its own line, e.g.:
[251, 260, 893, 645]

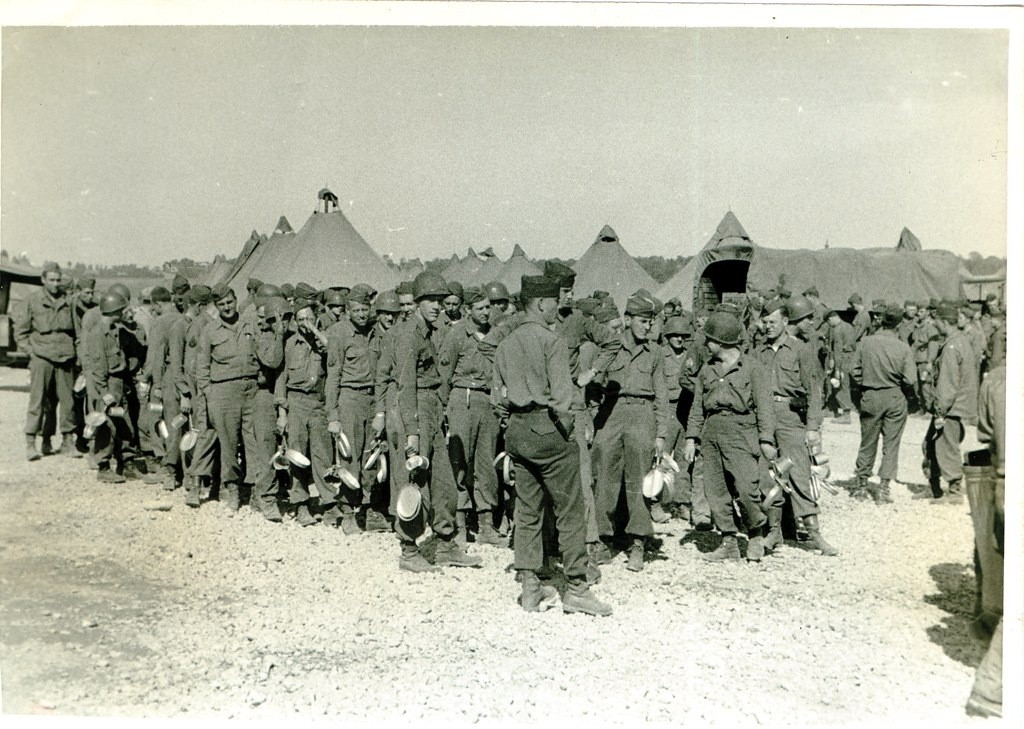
[964, 616, 1003, 719]
[12, 260, 1006, 638]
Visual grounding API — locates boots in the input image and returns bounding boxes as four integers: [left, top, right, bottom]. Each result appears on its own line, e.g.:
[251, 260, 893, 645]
[831, 412, 851, 424]
[26, 435, 40, 461]
[323, 506, 343, 527]
[796, 516, 839, 556]
[763, 507, 784, 549]
[822, 406, 834, 418]
[60, 433, 83, 458]
[702, 535, 740, 561]
[747, 527, 764, 560]
[679, 504, 690, 519]
[848, 476, 867, 500]
[366, 510, 392, 531]
[585, 541, 611, 585]
[478, 512, 509, 547]
[875, 478, 893, 505]
[454, 511, 466, 548]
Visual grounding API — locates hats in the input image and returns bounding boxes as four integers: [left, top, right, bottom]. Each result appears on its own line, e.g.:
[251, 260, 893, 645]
[281, 283, 295, 296]
[190, 284, 210, 303]
[576, 290, 620, 323]
[545, 260, 577, 287]
[779, 288, 791, 296]
[141, 286, 157, 300]
[77, 273, 96, 290]
[211, 282, 231, 300]
[626, 288, 664, 317]
[294, 296, 320, 314]
[463, 286, 487, 303]
[171, 274, 189, 289]
[802, 286, 1006, 324]
[750, 297, 761, 309]
[247, 278, 264, 289]
[759, 300, 784, 318]
[395, 282, 416, 293]
[296, 283, 321, 299]
[42, 262, 61, 273]
[510, 291, 521, 302]
[448, 282, 465, 297]
[521, 275, 561, 297]
[710, 304, 741, 319]
[347, 288, 370, 304]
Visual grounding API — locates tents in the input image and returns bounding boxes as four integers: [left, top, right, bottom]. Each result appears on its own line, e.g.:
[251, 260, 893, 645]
[196, 180, 1007, 314]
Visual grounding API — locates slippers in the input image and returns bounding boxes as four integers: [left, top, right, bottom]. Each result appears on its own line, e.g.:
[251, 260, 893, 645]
[627, 539, 644, 572]
[341, 511, 363, 535]
[651, 503, 671, 523]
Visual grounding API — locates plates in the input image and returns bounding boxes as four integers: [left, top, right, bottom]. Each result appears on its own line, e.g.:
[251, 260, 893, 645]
[338, 468, 360, 490]
[501, 455, 516, 486]
[364, 447, 387, 483]
[395, 483, 423, 522]
[337, 430, 352, 459]
[284, 448, 311, 469]
[806, 464, 839, 502]
[178, 429, 197, 452]
[82, 410, 106, 427]
[759, 470, 792, 511]
[640, 468, 675, 506]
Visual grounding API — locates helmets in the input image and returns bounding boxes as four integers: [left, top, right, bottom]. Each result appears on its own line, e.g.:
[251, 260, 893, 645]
[257, 284, 281, 296]
[698, 312, 741, 344]
[264, 297, 293, 320]
[108, 283, 131, 301]
[662, 317, 692, 338]
[100, 292, 127, 312]
[485, 281, 510, 300]
[329, 293, 346, 304]
[375, 291, 402, 311]
[353, 284, 377, 296]
[412, 272, 450, 300]
[786, 295, 815, 321]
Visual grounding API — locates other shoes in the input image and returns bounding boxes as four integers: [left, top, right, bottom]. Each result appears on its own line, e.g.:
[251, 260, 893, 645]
[399, 556, 444, 575]
[75, 439, 90, 452]
[910, 409, 933, 420]
[563, 592, 612, 615]
[41, 439, 51, 454]
[216, 484, 240, 517]
[97, 469, 126, 483]
[249, 486, 282, 522]
[518, 586, 556, 612]
[931, 485, 943, 498]
[966, 691, 1002, 716]
[435, 549, 483, 567]
[297, 506, 317, 527]
[143, 455, 213, 506]
[949, 480, 960, 493]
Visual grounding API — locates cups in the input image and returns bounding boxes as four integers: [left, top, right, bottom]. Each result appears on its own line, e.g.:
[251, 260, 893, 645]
[813, 451, 831, 466]
[147, 401, 162, 415]
[109, 403, 126, 417]
[405, 454, 431, 473]
[157, 419, 170, 439]
[271, 455, 290, 470]
[73, 375, 87, 392]
[170, 412, 188, 431]
[774, 453, 794, 475]
[829, 377, 839, 387]
[82, 425, 96, 439]
